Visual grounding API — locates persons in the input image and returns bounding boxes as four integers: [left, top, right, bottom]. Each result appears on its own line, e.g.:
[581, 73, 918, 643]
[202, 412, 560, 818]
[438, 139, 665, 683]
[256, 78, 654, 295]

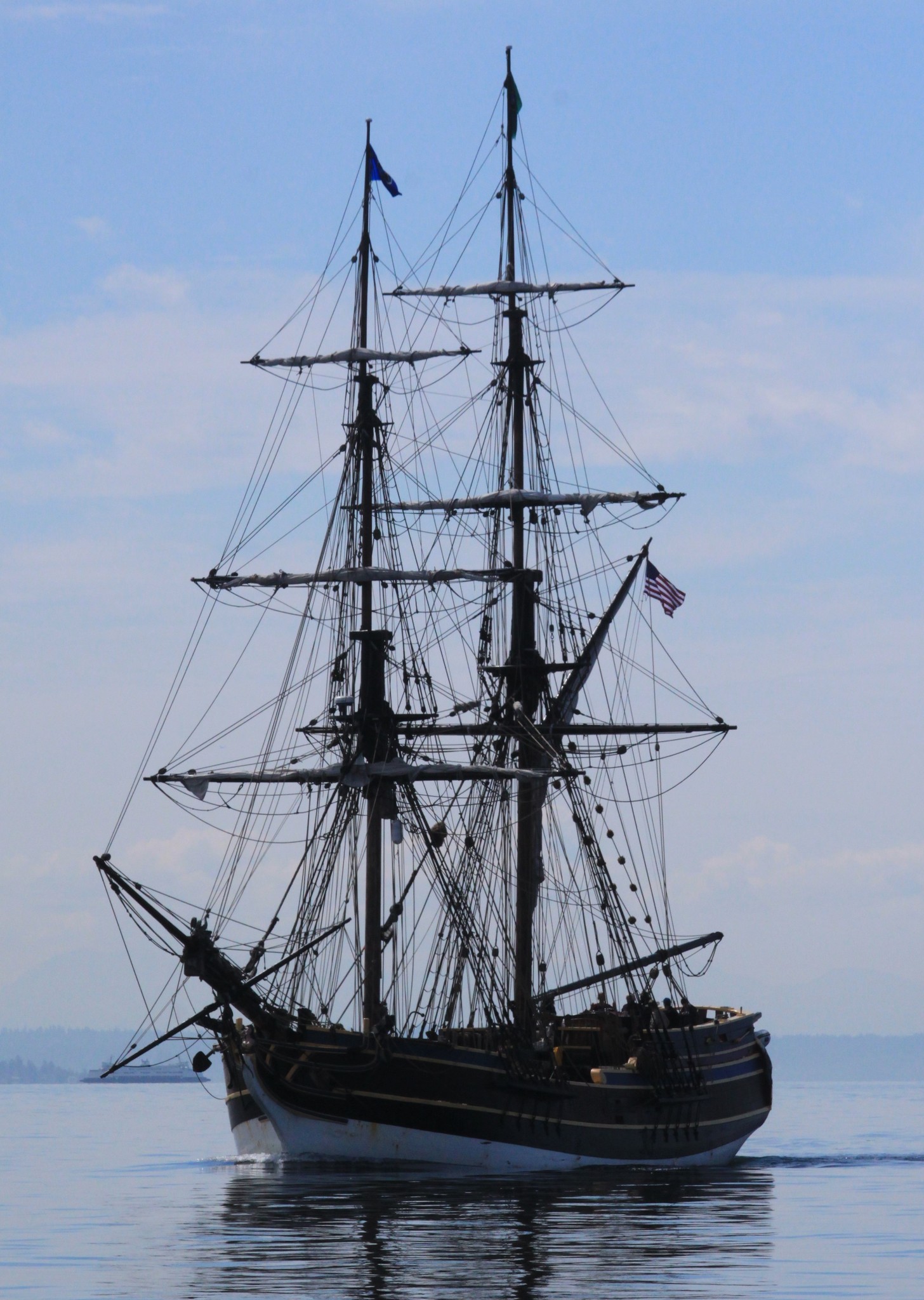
[621, 993, 640, 1031]
[680, 998, 700, 1025]
[639, 992, 652, 1016]
[648, 1000, 670, 1030]
[590, 992, 618, 1012]
[662, 998, 682, 1028]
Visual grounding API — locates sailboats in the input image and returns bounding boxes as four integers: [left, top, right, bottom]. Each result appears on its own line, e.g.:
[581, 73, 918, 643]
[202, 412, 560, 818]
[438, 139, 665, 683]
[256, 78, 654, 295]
[95, 45, 781, 1181]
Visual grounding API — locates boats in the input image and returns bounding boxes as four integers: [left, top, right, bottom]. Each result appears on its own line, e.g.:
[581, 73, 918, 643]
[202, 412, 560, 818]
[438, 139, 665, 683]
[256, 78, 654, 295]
[79, 1052, 212, 1084]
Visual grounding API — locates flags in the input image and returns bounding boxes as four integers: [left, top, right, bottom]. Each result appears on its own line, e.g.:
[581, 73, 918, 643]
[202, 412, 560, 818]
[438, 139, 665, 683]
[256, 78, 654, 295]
[368, 144, 404, 199]
[643, 559, 686, 620]
[505, 75, 524, 141]
